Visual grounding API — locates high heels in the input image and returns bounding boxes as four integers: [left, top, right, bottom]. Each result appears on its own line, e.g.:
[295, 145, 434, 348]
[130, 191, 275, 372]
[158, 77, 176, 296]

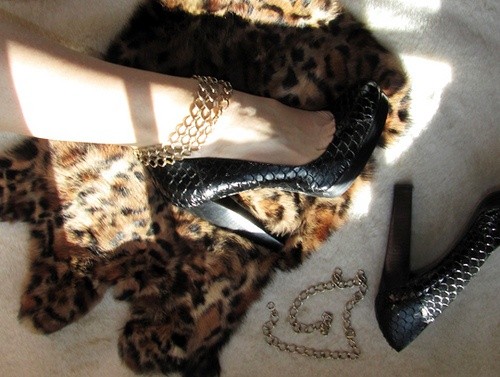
[374, 182, 498, 353]
[142, 84, 386, 251]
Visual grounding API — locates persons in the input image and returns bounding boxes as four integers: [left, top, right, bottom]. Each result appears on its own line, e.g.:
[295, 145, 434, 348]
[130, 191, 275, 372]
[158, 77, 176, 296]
[0, 7, 390, 254]
